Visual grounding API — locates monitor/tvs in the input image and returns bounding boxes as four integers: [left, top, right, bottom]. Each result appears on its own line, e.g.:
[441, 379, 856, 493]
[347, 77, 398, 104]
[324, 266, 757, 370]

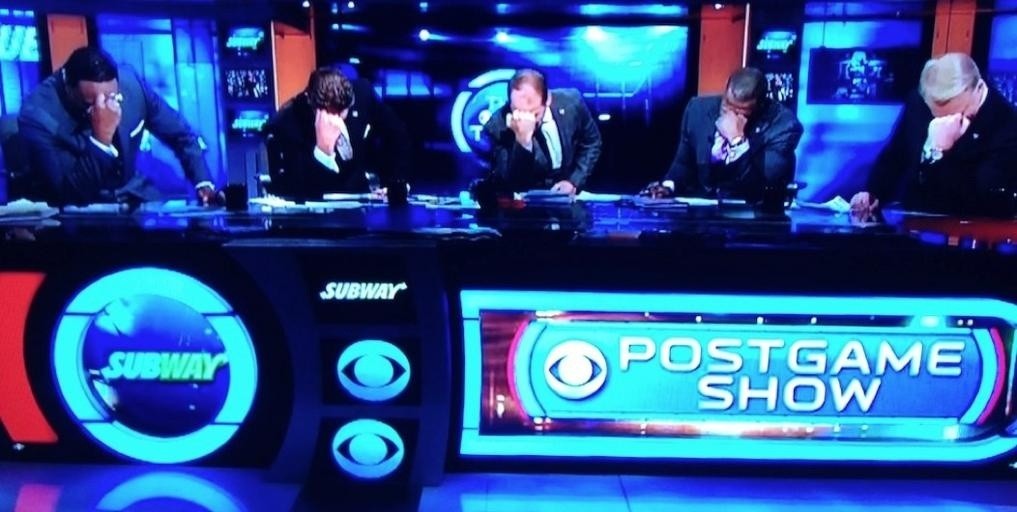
[807, 46, 921, 106]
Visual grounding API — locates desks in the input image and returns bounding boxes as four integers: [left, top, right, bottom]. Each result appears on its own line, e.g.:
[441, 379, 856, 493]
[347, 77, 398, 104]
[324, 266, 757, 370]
[1, 184, 1017, 482]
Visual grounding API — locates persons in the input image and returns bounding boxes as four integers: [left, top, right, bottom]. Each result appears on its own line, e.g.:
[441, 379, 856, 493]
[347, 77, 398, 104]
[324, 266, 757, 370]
[263, 67, 411, 200]
[484, 68, 603, 194]
[646, 67, 804, 213]
[849, 52, 1017, 214]
[18, 48, 215, 202]
